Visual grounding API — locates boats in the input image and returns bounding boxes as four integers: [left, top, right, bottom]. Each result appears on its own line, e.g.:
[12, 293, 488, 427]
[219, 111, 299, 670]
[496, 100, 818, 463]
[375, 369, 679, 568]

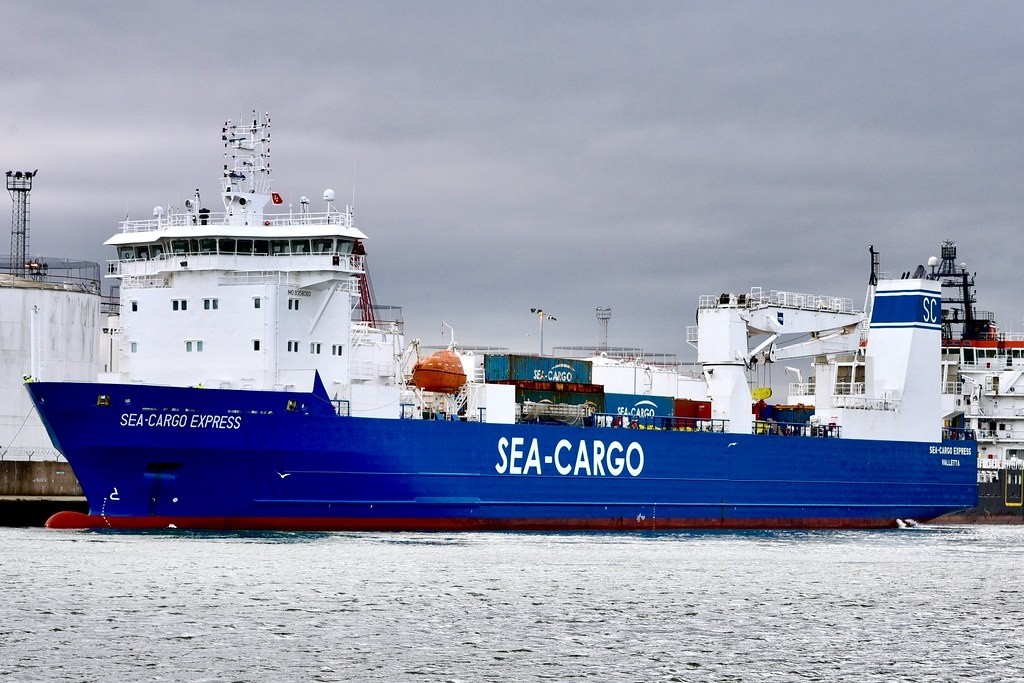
[22, 110, 979, 532]
[809, 238, 1024, 526]
